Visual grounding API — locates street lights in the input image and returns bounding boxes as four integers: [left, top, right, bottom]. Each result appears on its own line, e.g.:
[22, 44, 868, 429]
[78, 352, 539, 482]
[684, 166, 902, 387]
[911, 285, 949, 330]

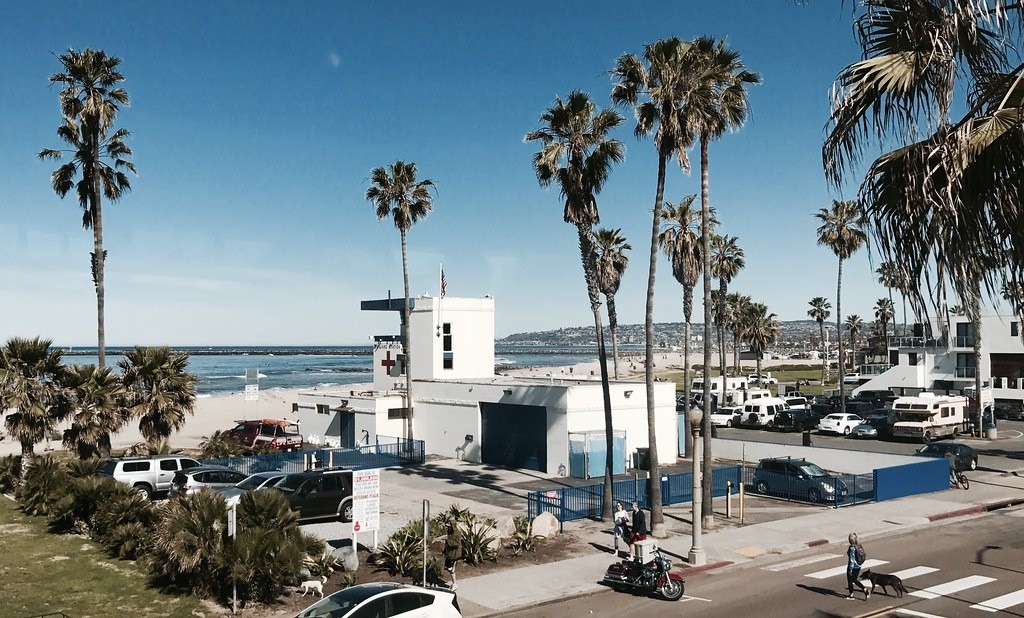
[825, 326, 830, 381]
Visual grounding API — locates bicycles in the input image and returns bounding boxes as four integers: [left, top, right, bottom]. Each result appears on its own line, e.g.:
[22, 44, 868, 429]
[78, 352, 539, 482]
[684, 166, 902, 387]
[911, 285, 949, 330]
[950, 470, 969, 492]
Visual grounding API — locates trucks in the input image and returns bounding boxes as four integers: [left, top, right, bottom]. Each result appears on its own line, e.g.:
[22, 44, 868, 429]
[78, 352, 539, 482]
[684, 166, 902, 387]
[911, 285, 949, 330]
[891, 391, 970, 444]
[691, 376, 748, 403]
[732, 388, 772, 406]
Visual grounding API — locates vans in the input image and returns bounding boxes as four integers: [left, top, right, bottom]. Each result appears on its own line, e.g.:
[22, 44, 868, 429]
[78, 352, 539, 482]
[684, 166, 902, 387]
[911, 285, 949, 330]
[740, 397, 790, 430]
[781, 397, 811, 409]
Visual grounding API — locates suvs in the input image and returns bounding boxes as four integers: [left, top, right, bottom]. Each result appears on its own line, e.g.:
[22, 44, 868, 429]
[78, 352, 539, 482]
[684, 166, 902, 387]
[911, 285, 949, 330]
[294, 581, 462, 618]
[837, 373, 860, 384]
[168, 467, 249, 499]
[746, 373, 778, 384]
[752, 456, 849, 502]
[775, 409, 820, 433]
[273, 468, 353, 523]
[98, 456, 202, 502]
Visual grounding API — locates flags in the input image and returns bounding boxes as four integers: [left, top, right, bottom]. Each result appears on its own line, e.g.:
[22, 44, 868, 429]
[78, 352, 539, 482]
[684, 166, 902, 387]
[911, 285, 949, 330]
[441, 269, 448, 299]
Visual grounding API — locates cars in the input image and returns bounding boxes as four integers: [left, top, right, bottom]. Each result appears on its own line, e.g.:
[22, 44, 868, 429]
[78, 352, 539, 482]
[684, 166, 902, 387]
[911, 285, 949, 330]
[817, 413, 864, 436]
[913, 443, 978, 471]
[216, 471, 291, 506]
[970, 413, 996, 436]
[677, 395, 694, 406]
[710, 406, 743, 428]
[775, 386, 896, 421]
[852, 416, 888, 439]
[790, 355, 800, 359]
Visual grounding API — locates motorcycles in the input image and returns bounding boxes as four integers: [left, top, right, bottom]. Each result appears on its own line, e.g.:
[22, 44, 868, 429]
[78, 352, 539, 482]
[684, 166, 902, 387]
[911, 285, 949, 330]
[605, 548, 685, 601]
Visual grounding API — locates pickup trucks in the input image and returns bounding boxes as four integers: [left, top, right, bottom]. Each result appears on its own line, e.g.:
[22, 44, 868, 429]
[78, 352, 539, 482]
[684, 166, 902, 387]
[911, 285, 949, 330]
[220, 418, 304, 457]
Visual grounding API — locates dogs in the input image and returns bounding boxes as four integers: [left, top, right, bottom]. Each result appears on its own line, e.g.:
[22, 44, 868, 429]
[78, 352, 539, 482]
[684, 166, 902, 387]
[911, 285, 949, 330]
[859, 569, 909, 598]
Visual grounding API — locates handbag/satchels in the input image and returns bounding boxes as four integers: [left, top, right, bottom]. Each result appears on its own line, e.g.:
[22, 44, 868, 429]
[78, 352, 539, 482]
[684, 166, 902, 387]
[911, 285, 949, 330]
[623, 519, 631, 543]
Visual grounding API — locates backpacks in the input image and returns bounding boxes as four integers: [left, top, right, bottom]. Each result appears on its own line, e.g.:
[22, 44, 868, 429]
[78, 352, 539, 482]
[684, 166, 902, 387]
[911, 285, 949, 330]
[851, 545, 866, 565]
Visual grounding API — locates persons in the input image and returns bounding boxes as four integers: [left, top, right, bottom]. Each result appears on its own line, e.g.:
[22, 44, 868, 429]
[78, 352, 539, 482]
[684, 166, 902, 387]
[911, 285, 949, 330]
[795, 380, 800, 391]
[611, 501, 631, 556]
[626, 502, 646, 561]
[561, 354, 668, 373]
[437, 519, 462, 591]
[944, 448, 962, 489]
[845, 532, 871, 600]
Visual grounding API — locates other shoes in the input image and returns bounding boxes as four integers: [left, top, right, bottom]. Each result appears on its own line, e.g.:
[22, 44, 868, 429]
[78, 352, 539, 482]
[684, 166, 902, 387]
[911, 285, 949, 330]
[448, 586, 451, 590]
[626, 557, 633, 561]
[451, 584, 458, 591]
[844, 596, 855, 600]
[865, 589, 871, 599]
